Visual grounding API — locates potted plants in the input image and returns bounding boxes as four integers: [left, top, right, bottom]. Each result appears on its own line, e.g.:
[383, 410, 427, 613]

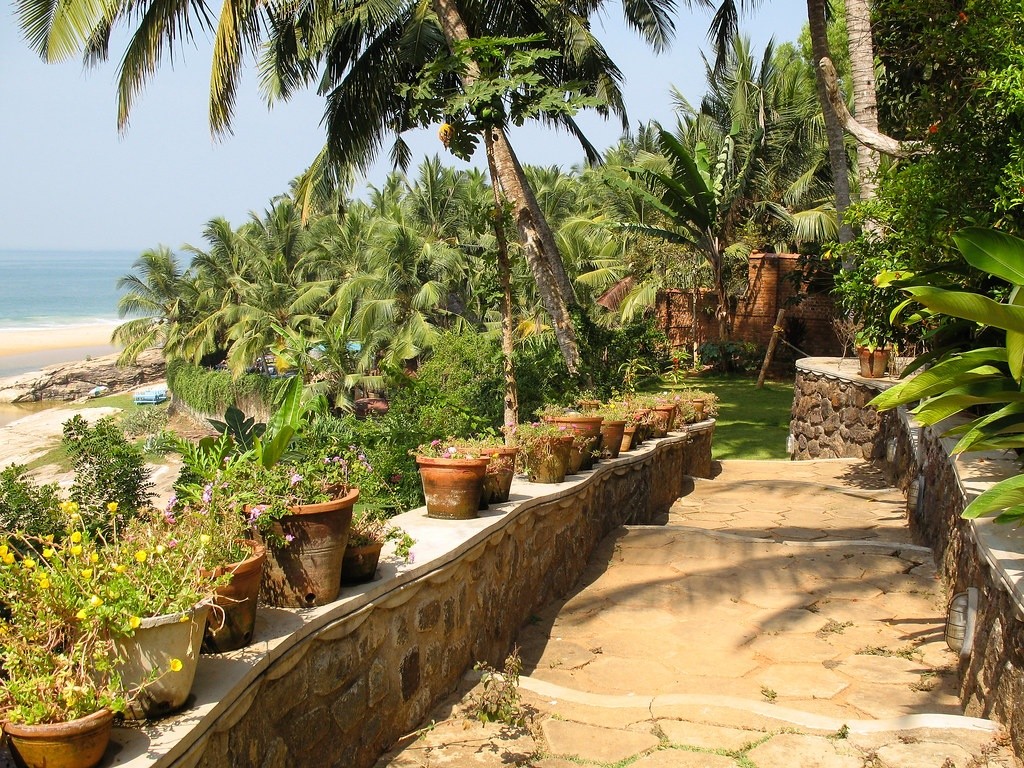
[344, 507, 415, 586]
[479, 458, 510, 509]
[857, 327, 897, 378]
[462, 426, 519, 503]
[536, 406, 603, 470]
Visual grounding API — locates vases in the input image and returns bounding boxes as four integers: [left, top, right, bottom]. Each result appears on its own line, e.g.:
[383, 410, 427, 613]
[0, 680, 112, 768]
[83, 595, 211, 720]
[241, 486, 359, 607]
[191, 539, 265, 654]
[525, 436, 574, 484]
[415, 455, 490, 519]
[599, 399, 707, 458]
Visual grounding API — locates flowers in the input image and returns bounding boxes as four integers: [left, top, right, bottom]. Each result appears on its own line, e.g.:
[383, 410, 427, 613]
[507, 422, 594, 450]
[411, 440, 480, 458]
[3, 434, 370, 683]
[601, 389, 716, 416]
